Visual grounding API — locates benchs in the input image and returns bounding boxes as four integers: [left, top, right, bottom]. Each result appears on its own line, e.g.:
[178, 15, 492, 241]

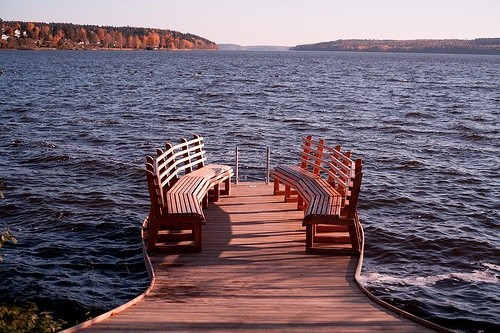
[145, 134, 235, 257]
[271, 134, 364, 255]
